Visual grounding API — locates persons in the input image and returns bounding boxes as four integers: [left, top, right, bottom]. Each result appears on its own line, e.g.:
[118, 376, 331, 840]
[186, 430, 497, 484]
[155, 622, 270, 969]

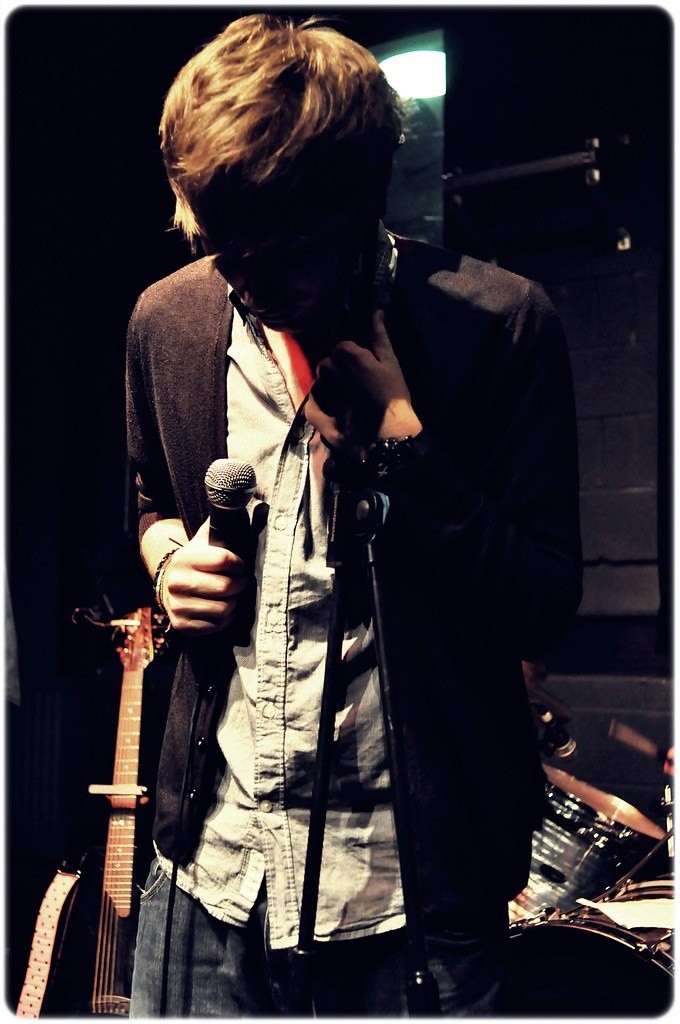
[124, 14, 586, 1018]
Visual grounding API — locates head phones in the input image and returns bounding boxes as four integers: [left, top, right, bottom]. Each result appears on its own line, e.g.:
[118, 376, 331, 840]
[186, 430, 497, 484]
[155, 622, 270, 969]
[336, 220, 398, 339]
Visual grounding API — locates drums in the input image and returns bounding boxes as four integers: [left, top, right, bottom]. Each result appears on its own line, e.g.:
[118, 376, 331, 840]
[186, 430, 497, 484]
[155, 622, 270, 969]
[503, 758, 673, 932]
[500, 872, 673, 1013]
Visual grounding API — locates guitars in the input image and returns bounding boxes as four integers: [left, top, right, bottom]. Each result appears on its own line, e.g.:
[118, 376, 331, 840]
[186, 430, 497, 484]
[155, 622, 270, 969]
[73, 599, 184, 1017]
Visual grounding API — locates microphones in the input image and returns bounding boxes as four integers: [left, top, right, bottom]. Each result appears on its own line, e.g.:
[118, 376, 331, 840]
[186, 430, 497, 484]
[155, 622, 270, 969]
[531, 699, 578, 761]
[195, 458, 257, 693]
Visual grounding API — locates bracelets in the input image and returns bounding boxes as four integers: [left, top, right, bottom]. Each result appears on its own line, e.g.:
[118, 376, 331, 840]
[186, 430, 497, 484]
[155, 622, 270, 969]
[372, 433, 415, 454]
[152, 549, 178, 615]
